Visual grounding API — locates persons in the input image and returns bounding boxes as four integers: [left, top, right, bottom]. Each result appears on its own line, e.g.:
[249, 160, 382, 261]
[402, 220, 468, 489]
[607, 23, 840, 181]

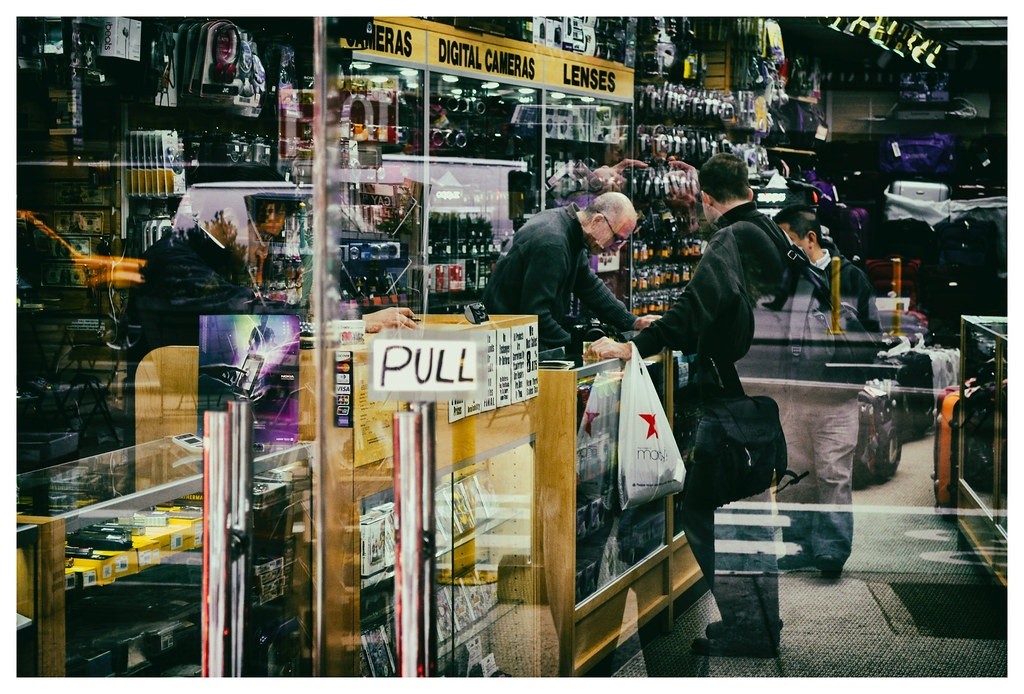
[479, 151, 882, 658]
[122, 163, 421, 487]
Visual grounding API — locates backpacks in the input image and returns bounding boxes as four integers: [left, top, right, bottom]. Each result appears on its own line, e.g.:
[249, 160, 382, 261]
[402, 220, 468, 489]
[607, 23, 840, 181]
[760, 245, 879, 405]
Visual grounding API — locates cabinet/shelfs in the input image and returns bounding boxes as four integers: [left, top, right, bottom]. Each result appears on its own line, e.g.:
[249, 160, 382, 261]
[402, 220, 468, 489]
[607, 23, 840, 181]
[17, 315, 705, 678]
[956, 314, 1007, 591]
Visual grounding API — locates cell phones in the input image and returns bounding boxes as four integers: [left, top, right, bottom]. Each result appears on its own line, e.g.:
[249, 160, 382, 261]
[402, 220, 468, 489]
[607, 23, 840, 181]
[233, 354, 265, 397]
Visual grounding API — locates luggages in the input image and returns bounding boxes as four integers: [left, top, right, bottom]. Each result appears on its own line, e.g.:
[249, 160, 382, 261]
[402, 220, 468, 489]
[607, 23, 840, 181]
[930, 384, 973, 507]
[830, 131, 1007, 489]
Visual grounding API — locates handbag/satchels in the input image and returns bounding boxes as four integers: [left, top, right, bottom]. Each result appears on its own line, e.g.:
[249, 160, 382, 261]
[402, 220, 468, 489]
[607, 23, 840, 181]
[899, 70, 953, 112]
[618, 342, 687, 511]
[679, 396, 789, 509]
[575, 366, 621, 498]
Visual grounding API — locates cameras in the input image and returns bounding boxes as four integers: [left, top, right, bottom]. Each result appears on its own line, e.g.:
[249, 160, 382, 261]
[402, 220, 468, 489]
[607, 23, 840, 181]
[571, 323, 608, 348]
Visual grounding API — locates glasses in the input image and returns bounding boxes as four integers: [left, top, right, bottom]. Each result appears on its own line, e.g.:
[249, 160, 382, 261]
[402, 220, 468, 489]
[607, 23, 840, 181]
[596, 210, 631, 245]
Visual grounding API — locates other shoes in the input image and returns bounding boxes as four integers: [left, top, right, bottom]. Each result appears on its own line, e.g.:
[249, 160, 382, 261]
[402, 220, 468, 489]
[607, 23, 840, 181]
[816, 554, 844, 578]
[690, 635, 781, 659]
[705, 620, 783, 639]
[777, 550, 815, 570]
